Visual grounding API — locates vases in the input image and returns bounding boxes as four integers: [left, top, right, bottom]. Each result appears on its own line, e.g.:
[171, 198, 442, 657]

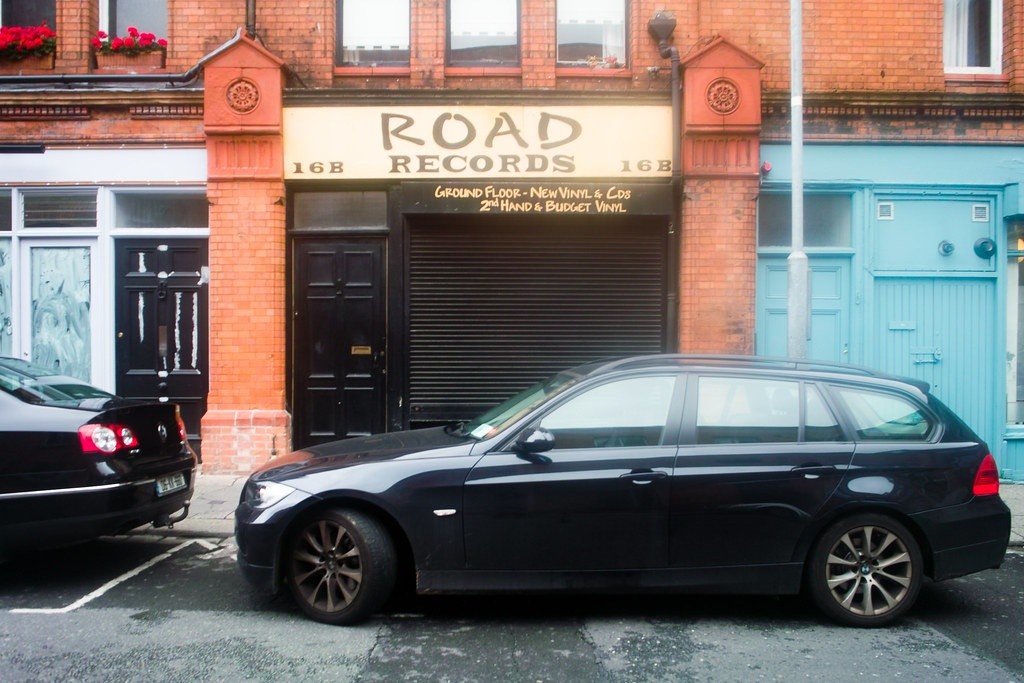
[96, 50, 165, 68]
[0, 52, 55, 69]
[606, 64, 616, 68]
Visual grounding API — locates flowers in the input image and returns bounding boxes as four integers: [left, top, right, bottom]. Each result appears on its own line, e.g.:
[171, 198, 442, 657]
[604, 55, 618, 64]
[587, 55, 598, 66]
[91, 26, 167, 57]
[0, 19, 57, 61]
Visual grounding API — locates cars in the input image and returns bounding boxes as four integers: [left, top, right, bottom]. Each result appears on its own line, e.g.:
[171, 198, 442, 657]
[233, 357, 1014, 630]
[1, 353, 198, 561]
